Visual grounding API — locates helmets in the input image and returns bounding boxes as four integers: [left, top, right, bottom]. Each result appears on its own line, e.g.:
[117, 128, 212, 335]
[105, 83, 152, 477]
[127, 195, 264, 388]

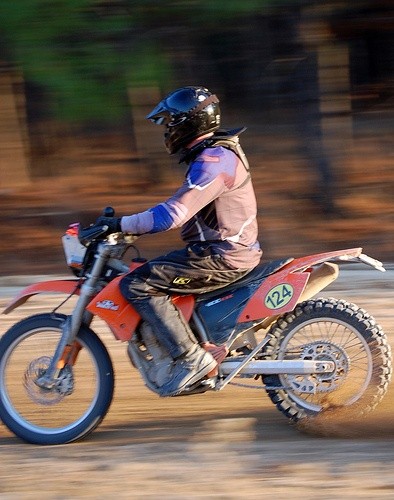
[145, 87, 221, 156]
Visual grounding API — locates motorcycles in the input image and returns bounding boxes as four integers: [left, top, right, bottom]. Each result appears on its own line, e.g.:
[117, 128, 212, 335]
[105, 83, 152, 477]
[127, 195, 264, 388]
[0, 205, 392, 447]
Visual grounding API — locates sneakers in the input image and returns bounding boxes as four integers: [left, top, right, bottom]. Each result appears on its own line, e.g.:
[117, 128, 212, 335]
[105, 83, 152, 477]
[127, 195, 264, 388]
[160, 346, 218, 397]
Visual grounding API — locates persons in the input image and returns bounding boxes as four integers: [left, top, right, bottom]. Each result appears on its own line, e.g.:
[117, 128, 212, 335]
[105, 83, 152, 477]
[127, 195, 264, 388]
[88, 86, 263, 398]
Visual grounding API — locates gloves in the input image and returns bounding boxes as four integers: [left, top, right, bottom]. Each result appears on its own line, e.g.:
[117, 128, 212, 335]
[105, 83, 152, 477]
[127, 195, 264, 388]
[96, 217, 121, 232]
